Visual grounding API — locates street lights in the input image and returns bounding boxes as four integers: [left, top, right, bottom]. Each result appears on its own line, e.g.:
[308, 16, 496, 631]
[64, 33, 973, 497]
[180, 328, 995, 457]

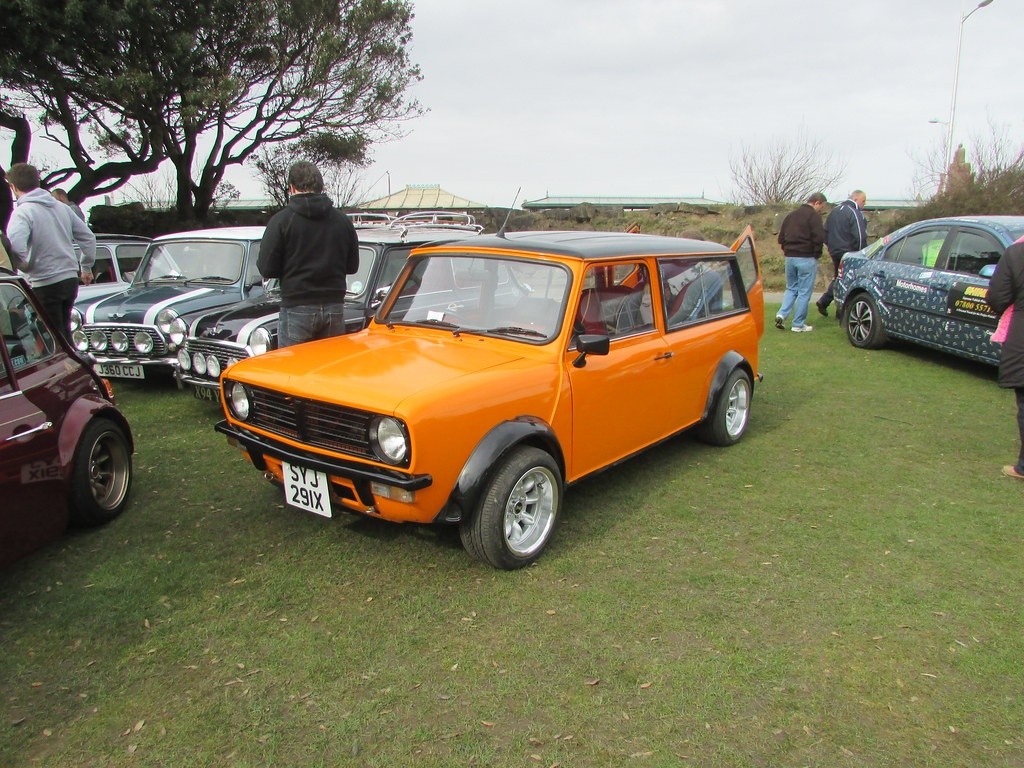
[940, 2, 996, 194]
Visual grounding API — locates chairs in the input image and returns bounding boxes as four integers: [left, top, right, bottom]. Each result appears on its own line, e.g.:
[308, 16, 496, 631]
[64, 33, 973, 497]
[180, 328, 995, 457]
[579, 286, 634, 335]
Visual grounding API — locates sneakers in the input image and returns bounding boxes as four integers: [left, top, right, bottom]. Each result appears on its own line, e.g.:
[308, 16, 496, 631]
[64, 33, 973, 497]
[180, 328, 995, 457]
[775, 314, 786, 330]
[791, 324, 813, 332]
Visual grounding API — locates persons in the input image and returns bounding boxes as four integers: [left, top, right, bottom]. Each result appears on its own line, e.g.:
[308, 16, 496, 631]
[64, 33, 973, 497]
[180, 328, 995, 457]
[255, 160, 361, 349]
[771, 192, 827, 333]
[984, 235, 1023, 483]
[3, 162, 98, 349]
[816, 189, 869, 319]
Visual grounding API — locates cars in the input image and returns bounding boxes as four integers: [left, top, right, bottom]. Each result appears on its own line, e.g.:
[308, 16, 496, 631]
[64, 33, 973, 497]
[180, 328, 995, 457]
[834, 213, 1024, 370]
[65, 214, 534, 408]
[0, 264, 133, 566]
[216, 223, 773, 570]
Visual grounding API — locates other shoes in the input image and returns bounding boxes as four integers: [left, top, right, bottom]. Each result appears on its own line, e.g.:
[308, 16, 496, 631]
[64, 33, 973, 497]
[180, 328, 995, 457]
[816, 301, 828, 317]
[1004, 464, 1023, 478]
[836, 311, 841, 319]
